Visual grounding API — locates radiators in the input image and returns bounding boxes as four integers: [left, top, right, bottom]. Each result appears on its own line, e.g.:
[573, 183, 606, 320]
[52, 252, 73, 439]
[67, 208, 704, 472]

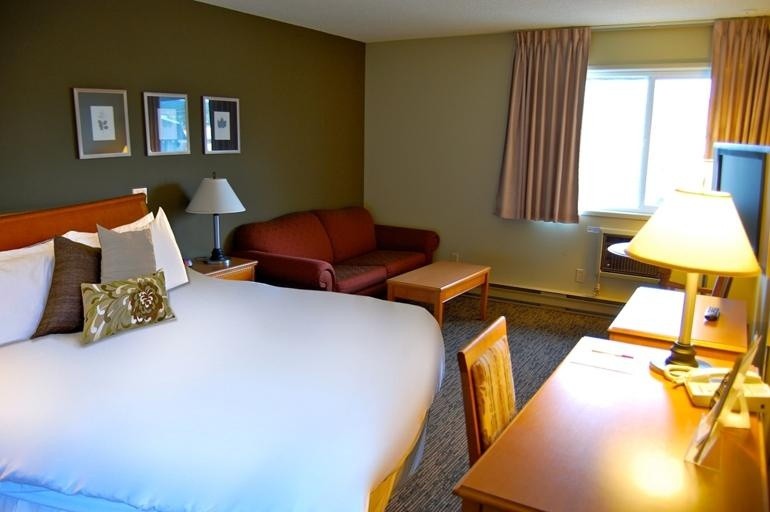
[595, 228, 672, 293]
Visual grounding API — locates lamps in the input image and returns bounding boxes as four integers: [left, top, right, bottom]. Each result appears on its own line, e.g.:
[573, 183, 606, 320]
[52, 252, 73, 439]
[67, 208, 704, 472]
[185, 173, 245, 266]
[624, 190, 763, 383]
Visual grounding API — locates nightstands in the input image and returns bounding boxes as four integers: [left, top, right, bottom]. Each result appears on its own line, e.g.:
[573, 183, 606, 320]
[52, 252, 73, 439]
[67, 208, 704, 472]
[183, 255, 259, 282]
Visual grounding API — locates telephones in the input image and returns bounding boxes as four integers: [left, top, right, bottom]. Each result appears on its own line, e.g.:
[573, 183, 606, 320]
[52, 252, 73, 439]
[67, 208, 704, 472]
[683, 368, 770, 412]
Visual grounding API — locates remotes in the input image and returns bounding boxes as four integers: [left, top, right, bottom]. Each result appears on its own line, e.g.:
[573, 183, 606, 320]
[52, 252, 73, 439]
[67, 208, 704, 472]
[704, 305, 720, 320]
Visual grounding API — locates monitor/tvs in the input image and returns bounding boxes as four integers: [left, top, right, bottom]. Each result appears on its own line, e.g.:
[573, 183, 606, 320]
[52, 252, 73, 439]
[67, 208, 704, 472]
[711, 141, 770, 272]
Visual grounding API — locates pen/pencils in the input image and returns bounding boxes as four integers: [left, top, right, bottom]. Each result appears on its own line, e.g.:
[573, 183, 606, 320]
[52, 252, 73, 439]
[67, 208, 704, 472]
[593, 349, 633, 358]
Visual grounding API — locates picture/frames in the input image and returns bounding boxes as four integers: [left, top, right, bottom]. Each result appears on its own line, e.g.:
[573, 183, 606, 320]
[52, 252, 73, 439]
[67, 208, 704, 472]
[143, 91, 191, 156]
[72, 88, 132, 159]
[202, 96, 241, 154]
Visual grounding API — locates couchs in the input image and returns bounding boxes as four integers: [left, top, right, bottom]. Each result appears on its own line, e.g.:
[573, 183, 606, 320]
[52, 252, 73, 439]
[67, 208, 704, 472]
[228, 206, 440, 297]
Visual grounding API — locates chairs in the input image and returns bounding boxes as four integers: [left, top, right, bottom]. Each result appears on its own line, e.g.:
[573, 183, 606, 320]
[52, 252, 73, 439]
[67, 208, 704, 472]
[457, 315, 517, 469]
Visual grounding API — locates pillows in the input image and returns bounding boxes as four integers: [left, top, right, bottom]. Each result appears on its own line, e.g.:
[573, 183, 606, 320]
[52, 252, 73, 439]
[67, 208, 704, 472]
[95, 224, 155, 283]
[29, 234, 101, 339]
[129, 206, 189, 293]
[61, 211, 154, 239]
[81, 268, 176, 347]
[0, 237, 54, 346]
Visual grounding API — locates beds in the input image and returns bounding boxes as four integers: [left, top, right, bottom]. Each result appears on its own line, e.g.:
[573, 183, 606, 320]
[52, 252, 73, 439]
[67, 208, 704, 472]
[1, 193, 446, 512]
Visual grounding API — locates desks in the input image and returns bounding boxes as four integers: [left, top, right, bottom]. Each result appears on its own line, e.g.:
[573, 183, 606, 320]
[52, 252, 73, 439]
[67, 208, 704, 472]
[453, 334, 769, 512]
[607, 286, 748, 364]
